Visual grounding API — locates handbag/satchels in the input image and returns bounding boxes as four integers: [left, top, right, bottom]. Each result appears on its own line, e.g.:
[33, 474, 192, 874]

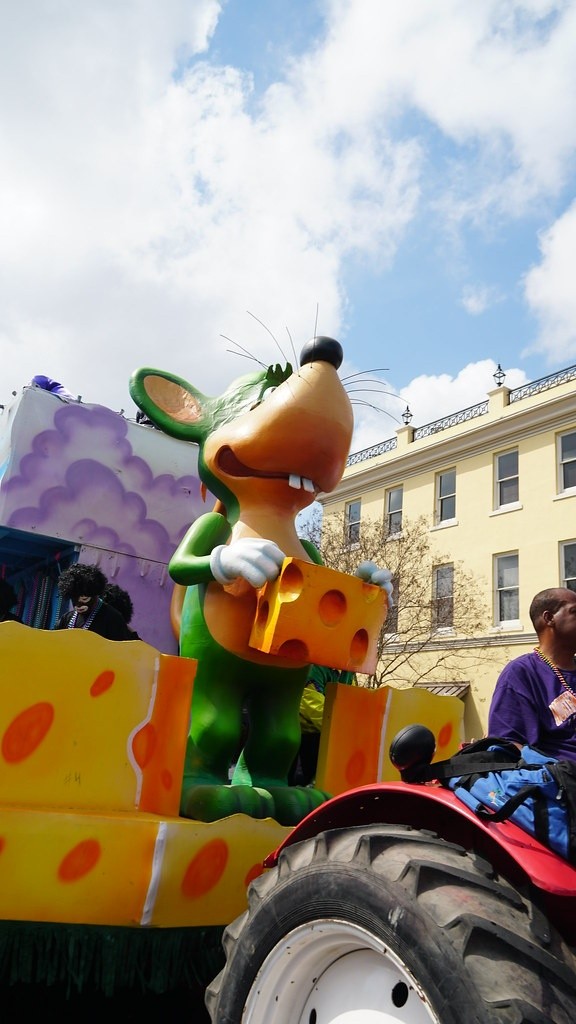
[404, 737, 576, 869]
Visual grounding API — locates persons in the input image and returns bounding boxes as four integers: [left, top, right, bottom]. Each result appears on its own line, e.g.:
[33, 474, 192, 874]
[288, 666, 364, 787]
[487, 587, 576, 764]
[100, 584, 142, 641]
[0, 577, 24, 623]
[53, 563, 129, 641]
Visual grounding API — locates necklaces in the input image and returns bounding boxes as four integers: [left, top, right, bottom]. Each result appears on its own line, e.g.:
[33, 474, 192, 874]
[68, 599, 103, 629]
[535, 647, 576, 697]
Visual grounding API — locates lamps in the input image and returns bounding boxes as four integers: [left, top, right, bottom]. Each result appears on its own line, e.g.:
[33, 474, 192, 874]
[401, 405, 413, 425]
[493, 363, 506, 387]
